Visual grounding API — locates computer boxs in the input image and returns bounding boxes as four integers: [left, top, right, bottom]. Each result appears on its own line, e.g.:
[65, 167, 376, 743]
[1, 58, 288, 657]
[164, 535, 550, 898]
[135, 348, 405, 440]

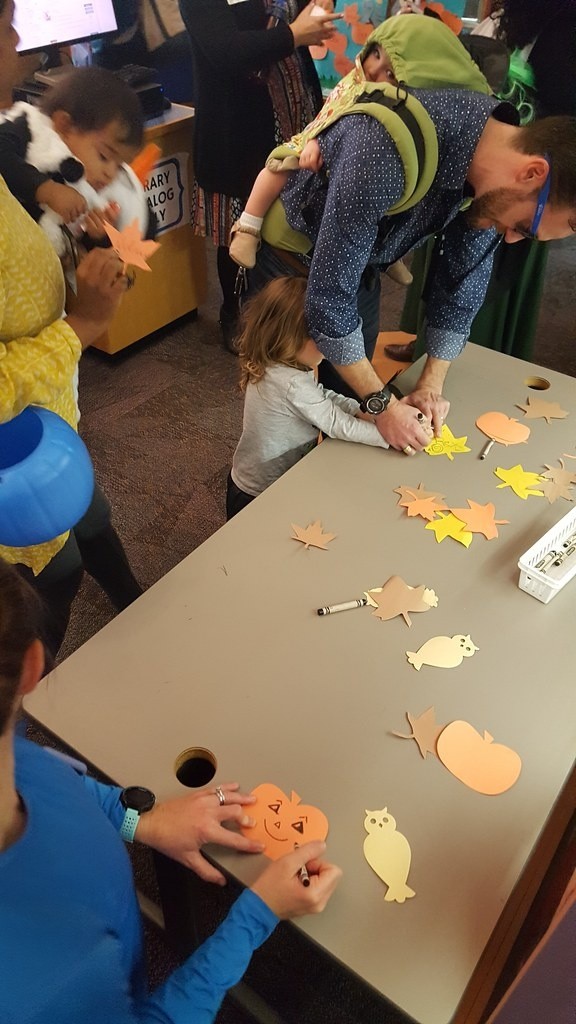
[12, 83, 163, 123]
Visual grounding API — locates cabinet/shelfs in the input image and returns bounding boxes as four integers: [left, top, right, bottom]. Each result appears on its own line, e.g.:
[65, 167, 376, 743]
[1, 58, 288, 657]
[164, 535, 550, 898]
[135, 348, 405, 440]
[60, 101, 209, 355]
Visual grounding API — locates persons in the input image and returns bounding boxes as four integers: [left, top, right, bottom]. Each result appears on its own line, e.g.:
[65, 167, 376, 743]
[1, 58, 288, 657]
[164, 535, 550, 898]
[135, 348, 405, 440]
[229, 15, 499, 285]
[0, 0, 146, 677]
[178, 0, 344, 358]
[0, 553, 342, 1024]
[383, 0, 576, 362]
[227, 275, 390, 519]
[235, 84, 576, 440]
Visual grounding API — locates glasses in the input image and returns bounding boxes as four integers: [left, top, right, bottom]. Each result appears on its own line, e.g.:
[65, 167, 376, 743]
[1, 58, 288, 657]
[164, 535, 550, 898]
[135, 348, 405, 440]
[513, 152, 551, 242]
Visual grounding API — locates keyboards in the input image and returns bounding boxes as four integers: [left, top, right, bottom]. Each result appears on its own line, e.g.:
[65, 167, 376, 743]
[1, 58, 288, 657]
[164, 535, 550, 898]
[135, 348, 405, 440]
[107, 62, 158, 88]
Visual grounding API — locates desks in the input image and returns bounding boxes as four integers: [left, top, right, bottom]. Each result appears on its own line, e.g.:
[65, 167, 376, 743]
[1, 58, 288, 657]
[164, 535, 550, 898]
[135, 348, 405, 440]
[19, 341, 575, 1023]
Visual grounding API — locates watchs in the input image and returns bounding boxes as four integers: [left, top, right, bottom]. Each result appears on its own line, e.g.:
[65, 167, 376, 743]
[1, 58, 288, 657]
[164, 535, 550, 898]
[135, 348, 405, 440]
[359, 384, 392, 415]
[118, 786, 156, 844]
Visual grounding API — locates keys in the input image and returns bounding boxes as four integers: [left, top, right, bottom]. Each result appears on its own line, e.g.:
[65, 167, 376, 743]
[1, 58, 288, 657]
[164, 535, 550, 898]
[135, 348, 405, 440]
[234, 265, 248, 295]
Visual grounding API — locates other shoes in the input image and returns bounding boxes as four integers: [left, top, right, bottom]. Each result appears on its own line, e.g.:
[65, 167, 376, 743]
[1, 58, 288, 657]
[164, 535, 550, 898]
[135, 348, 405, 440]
[385, 261, 413, 286]
[229, 222, 260, 269]
[217, 303, 239, 357]
[385, 339, 416, 363]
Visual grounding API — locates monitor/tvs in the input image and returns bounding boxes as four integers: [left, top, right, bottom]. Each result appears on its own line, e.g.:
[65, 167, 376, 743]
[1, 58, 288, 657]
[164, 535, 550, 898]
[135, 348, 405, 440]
[11, 0, 119, 89]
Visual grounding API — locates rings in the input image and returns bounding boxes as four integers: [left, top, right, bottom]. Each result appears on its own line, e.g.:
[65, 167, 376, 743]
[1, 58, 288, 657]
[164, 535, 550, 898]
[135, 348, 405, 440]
[440, 416, 444, 420]
[215, 786, 226, 805]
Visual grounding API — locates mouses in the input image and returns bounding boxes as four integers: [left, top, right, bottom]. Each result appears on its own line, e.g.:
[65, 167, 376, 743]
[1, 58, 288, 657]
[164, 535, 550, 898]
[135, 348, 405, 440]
[163, 97, 172, 109]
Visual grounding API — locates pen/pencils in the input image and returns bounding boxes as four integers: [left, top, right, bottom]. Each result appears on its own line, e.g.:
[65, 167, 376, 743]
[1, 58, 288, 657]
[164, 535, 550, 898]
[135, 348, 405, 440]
[417, 413, 424, 424]
[480, 438, 495, 460]
[318, 598, 367, 615]
[527, 531, 576, 580]
[294, 841, 310, 887]
